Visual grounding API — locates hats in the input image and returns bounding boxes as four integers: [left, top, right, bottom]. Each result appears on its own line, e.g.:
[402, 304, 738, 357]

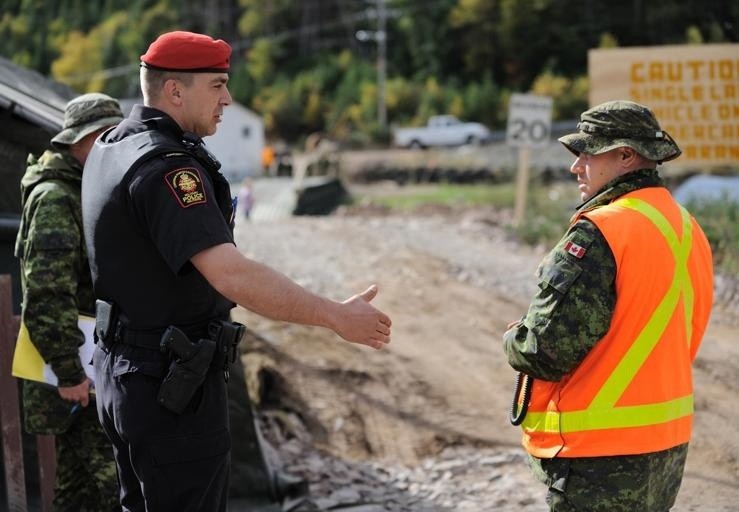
[557, 100, 681, 165]
[50, 92, 124, 150]
[139, 30, 232, 73]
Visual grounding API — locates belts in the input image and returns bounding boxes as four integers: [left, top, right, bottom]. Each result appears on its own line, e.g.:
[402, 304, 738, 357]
[113, 325, 162, 351]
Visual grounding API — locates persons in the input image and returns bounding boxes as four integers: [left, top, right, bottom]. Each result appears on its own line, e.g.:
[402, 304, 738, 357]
[82, 31, 391, 512]
[505, 100, 715, 512]
[239, 179, 254, 218]
[14, 92, 125, 512]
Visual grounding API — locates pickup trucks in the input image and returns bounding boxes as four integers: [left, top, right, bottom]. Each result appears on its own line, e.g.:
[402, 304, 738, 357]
[393, 113, 492, 149]
